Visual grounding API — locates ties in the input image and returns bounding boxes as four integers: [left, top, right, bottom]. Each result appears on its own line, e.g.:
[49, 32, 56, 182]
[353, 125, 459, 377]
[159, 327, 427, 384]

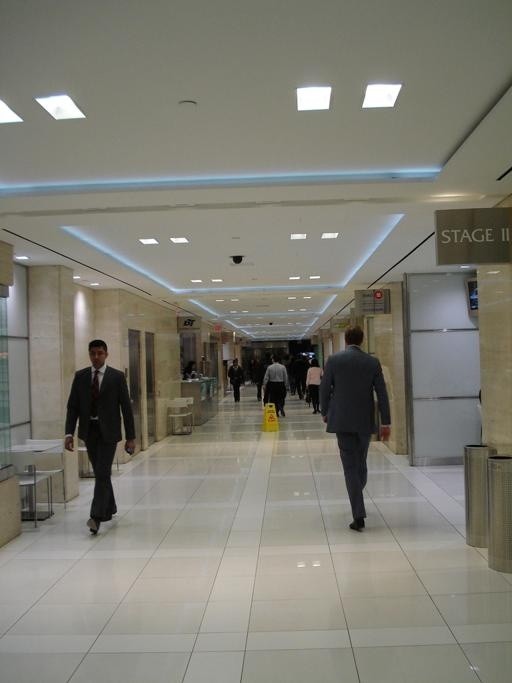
[92, 370, 100, 401]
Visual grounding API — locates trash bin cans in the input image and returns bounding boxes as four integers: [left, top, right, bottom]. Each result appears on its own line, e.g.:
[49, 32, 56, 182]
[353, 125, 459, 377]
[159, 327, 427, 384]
[463, 445, 512, 573]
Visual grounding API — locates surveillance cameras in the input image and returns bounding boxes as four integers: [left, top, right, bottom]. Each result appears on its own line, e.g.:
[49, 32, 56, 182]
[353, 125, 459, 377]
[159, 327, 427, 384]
[269, 323, 273, 325]
[233, 256, 242, 264]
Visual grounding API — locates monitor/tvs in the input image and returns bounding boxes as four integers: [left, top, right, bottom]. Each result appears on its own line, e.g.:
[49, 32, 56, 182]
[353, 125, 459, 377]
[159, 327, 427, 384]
[464, 277, 478, 316]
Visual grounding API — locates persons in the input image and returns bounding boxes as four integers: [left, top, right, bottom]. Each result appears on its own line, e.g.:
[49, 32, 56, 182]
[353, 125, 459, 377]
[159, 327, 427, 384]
[198, 354, 211, 379]
[63, 338, 136, 537]
[182, 360, 196, 380]
[247, 350, 310, 416]
[316, 324, 392, 531]
[228, 358, 245, 402]
[305, 358, 324, 414]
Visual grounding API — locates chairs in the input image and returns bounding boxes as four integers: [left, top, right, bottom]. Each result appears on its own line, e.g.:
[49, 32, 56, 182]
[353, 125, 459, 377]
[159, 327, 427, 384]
[167, 397, 195, 435]
[2, 437, 68, 529]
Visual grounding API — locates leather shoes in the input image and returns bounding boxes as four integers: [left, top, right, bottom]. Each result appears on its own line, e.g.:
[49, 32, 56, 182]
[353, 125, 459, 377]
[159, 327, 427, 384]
[86, 517, 101, 535]
[349, 518, 366, 530]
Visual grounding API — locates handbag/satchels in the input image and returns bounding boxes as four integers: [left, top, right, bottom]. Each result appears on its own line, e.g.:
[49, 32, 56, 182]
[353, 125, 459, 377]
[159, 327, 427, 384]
[305, 388, 313, 403]
